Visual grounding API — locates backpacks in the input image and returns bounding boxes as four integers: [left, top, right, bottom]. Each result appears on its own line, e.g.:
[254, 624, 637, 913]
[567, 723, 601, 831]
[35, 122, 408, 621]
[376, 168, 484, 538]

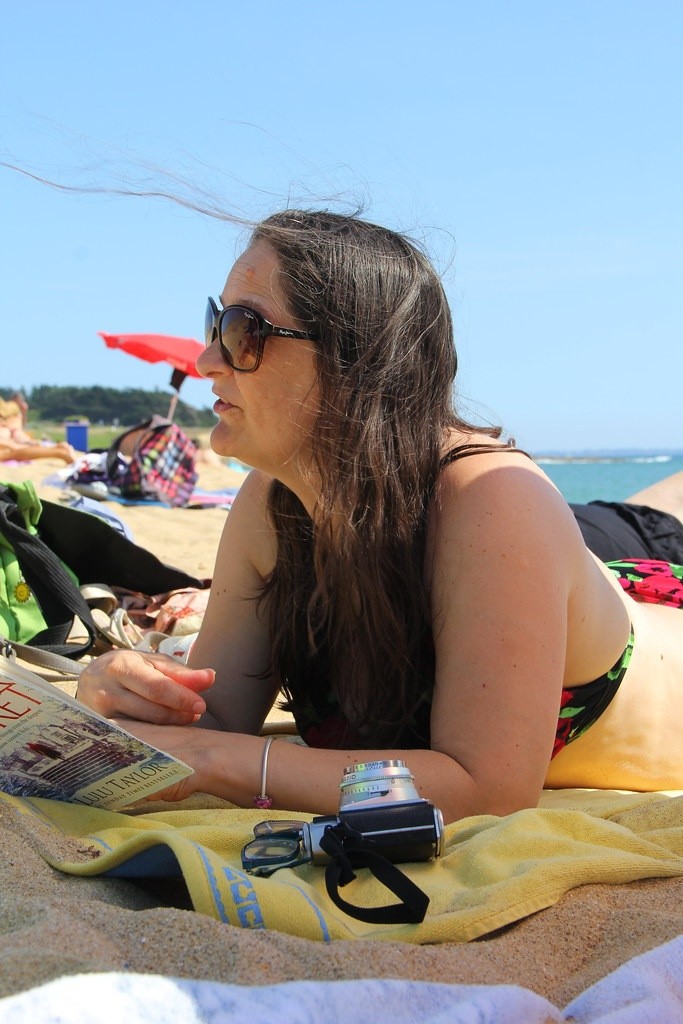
[0, 477, 95, 659]
[108, 411, 198, 508]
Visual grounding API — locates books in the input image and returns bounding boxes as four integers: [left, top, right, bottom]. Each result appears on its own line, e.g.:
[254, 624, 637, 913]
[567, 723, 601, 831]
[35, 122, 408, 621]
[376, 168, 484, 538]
[0, 655, 194, 810]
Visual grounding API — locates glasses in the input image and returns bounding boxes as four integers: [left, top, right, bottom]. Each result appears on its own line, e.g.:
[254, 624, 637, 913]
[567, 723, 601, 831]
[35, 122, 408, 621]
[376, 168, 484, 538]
[205, 296, 322, 374]
[241, 820, 312, 879]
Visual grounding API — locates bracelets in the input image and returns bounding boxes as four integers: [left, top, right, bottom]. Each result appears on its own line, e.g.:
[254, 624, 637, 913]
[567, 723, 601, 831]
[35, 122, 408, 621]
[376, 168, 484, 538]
[255, 737, 272, 809]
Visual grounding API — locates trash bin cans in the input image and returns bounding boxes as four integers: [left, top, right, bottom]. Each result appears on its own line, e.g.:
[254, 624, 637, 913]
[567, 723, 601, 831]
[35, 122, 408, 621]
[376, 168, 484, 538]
[64, 416, 89, 452]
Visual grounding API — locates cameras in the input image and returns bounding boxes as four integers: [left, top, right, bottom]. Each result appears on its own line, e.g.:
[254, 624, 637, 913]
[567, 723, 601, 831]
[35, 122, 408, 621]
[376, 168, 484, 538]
[302, 759, 445, 867]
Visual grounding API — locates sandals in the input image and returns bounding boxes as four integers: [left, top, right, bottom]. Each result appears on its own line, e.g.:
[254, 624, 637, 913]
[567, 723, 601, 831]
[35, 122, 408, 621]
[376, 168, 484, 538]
[91, 606, 156, 657]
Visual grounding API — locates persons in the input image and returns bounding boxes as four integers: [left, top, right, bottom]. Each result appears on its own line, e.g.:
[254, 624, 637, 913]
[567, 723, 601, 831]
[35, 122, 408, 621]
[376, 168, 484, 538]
[0, 392, 74, 465]
[73, 209, 683, 825]
[568, 471, 683, 566]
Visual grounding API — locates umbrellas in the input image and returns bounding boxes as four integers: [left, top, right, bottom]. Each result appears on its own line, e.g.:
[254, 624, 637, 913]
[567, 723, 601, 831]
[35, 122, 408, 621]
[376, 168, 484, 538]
[96, 331, 206, 420]
